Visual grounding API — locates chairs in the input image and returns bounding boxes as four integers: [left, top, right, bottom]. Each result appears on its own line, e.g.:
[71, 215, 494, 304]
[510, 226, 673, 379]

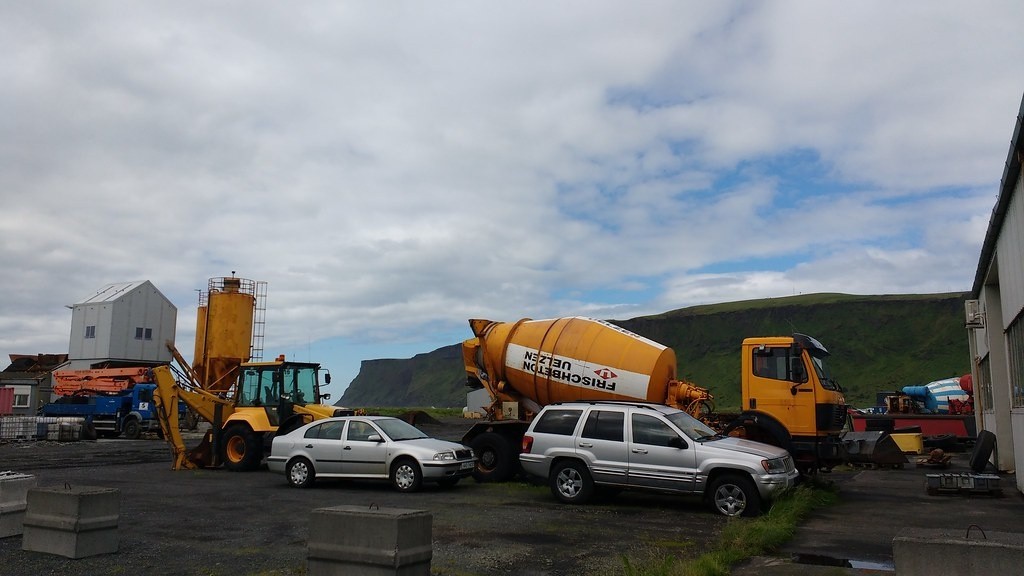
[265, 386, 275, 404]
[791, 359, 802, 382]
[649, 421, 669, 446]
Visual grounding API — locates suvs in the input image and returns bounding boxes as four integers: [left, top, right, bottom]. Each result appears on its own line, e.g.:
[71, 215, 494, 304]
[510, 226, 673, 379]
[519, 399, 799, 522]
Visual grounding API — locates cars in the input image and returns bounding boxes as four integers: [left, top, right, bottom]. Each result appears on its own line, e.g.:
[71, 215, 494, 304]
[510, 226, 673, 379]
[265, 415, 480, 495]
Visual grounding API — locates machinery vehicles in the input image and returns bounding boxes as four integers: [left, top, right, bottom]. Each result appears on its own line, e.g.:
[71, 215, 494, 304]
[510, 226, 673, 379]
[150, 355, 370, 473]
[32, 366, 186, 440]
[874, 373, 972, 413]
[457, 314, 851, 485]
